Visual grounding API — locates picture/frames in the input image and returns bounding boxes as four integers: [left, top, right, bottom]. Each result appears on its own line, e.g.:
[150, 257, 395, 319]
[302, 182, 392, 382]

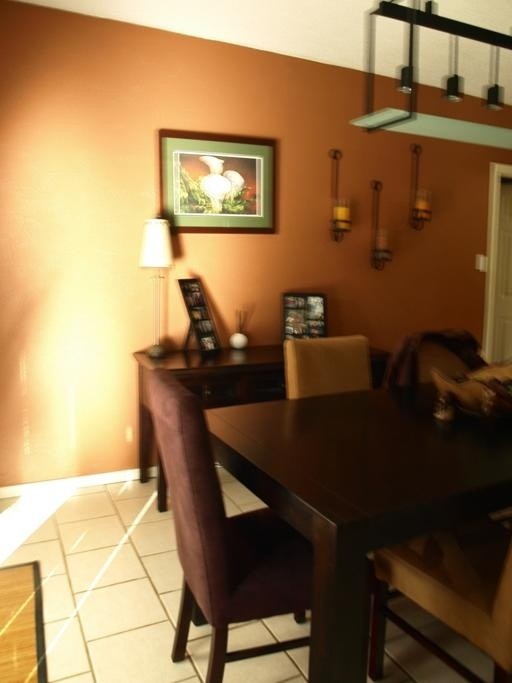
[178, 278, 222, 356]
[155, 128, 276, 234]
[282, 292, 327, 340]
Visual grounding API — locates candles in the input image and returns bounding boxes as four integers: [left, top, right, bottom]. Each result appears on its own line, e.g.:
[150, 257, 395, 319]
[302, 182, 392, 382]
[414, 201, 432, 220]
[332, 206, 351, 230]
[375, 230, 389, 251]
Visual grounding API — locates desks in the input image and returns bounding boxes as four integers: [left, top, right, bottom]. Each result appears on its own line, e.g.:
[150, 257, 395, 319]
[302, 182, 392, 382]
[132, 344, 389, 512]
[202, 382, 512, 683]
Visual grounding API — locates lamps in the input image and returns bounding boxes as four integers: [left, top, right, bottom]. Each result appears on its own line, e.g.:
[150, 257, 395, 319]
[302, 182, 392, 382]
[349, 0, 511, 151]
[137, 218, 178, 359]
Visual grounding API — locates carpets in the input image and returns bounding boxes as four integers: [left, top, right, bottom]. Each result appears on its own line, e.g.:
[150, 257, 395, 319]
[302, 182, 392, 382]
[0, 560, 47, 683]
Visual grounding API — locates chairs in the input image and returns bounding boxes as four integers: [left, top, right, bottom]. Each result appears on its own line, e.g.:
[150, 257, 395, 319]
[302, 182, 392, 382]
[141, 368, 389, 683]
[283, 334, 374, 401]
[368, 514, 512, 683]
